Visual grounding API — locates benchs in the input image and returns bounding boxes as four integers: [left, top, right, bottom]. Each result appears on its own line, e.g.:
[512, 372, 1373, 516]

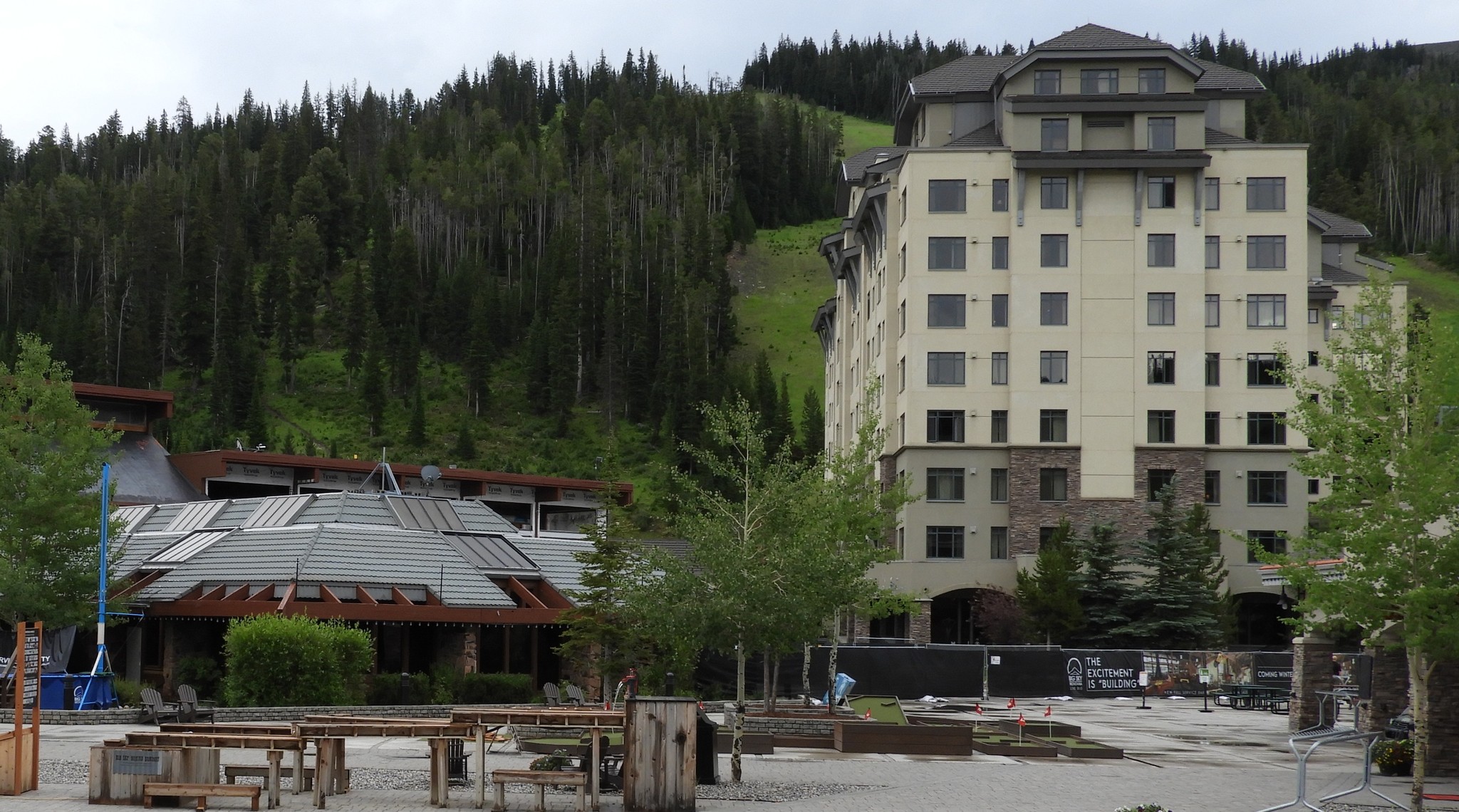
[1212, 692, 1290, 714]
[143, 782, 262, 812]
[225, 766, 350, 793]
[493, 769, 588, 812]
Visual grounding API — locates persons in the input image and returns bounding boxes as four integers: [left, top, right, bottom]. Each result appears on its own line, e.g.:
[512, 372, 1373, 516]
[1332, 655, 1342, 689]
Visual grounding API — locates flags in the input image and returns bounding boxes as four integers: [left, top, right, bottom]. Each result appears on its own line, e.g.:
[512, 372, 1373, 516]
[1044, 707, 1050, 717]
[865, 709, 870, 719]
[1017, 715, 1026, 727]
[1008, 699, 1016, 708]
[976, 705, 982, 714]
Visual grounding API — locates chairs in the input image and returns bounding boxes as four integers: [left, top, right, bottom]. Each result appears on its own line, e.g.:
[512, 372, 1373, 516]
[553, 736, 624, 793]
[447, 739, 472, 785]
[140, 684, 216, 726]
[544, 683, 600, 707]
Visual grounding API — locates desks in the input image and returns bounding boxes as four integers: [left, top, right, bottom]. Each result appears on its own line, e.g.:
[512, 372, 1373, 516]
[1221, 684, 1266, 704]
[1241, 687, 1284, 708]
